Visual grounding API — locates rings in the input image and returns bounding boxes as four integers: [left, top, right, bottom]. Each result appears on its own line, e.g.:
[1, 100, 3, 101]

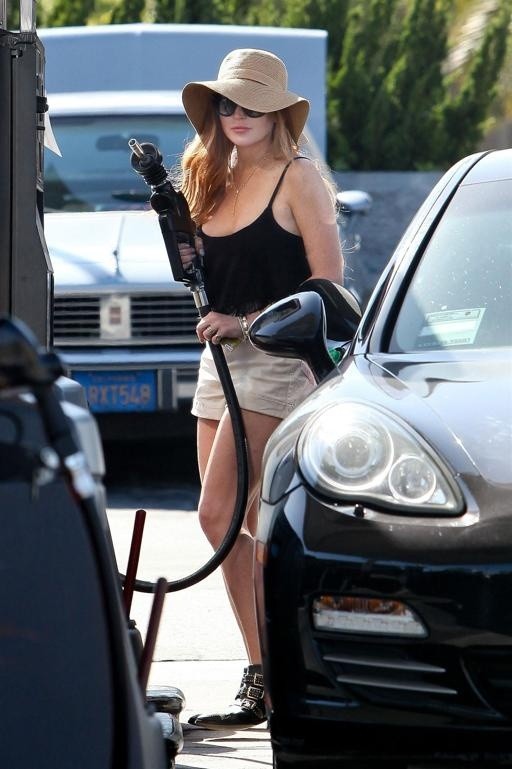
[206, 326, 214, 334]
[215, 334, 222, 342]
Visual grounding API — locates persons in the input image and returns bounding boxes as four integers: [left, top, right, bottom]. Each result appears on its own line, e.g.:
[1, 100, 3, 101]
[175, 47, 345, 732]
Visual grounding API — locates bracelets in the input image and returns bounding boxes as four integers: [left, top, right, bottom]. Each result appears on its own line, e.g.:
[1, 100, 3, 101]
[238, 314, 250, 341]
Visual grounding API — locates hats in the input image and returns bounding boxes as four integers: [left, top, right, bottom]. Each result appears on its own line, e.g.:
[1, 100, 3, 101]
[180, 48, 311, 151]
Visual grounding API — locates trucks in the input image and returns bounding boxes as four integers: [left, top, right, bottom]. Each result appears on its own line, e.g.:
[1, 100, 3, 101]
[29, 22, 368, 441]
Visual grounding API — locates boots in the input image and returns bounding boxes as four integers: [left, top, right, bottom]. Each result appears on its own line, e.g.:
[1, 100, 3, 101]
[187, 663, 268, 732]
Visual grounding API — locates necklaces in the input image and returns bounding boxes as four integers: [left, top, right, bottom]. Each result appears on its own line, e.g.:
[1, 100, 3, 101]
[230, 154, 270, 216]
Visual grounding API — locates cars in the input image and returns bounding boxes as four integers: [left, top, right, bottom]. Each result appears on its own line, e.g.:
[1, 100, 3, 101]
[246, 148, 511, 769]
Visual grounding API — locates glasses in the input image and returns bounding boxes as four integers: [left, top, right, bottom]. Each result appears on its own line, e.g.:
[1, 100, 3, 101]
[215, 91, 266, 118]
